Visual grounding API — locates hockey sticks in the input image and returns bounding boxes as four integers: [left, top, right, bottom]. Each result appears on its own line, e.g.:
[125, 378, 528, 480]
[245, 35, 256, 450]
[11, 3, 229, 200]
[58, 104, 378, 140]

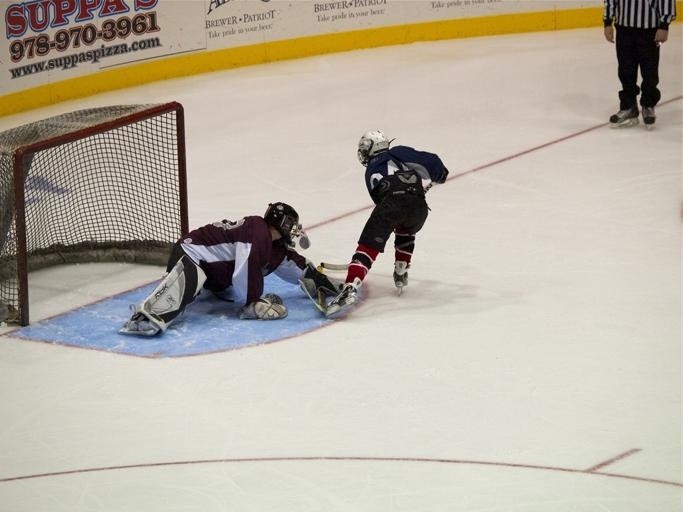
[321, 181, 437, 271]
[298, 264, 328, 314]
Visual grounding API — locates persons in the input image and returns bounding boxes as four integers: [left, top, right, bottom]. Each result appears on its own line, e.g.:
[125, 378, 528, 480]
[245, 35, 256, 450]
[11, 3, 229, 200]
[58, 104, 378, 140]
[327, 130, 449, 307]
[604, 0, 676, 121]
[117, 202, 343, 335]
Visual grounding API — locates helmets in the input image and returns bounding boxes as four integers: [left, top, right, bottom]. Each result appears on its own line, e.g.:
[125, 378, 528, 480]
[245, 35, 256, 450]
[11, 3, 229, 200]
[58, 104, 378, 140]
[357, 130, 396, 167]
[263, 202, 303, 247]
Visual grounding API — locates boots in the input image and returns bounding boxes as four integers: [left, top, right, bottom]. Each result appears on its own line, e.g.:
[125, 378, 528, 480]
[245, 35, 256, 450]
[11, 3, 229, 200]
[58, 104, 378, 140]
[325, 276, 363, 316]
[393, 260, 408, 287]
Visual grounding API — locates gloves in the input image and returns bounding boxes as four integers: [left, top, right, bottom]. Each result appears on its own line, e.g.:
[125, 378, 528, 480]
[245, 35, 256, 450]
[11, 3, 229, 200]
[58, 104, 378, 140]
[300, 261, 339, 315]
[236, 298, 288, 320]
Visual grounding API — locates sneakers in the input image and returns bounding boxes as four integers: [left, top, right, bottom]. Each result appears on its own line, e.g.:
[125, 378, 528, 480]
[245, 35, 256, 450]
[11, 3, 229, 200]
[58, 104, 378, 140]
[642, 105, 655, 124]
[127, 312, 153, 332]
[610, 102, 639, 124]
